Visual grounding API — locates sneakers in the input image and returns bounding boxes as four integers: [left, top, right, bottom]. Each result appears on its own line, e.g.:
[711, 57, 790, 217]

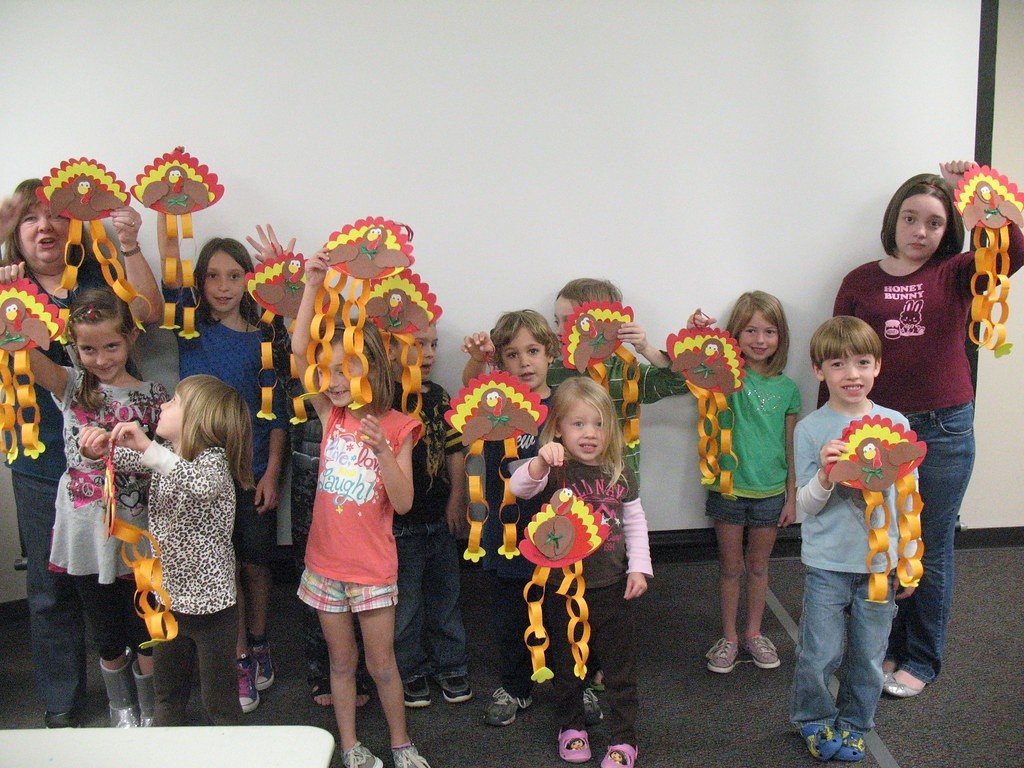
[483, 687, 533, 725]
[583, 688, 604, 726]
[705, 638, 739, 673]
[740, 634, 781, 668]
[251, 643, 275, 690]
[236, 654, 259, 714]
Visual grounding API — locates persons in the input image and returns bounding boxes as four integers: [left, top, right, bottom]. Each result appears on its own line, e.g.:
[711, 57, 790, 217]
[683, 290, 798, 673]
[372, 299, 474, 708]
[793, 316, 922, 760]
[0, 178, 369, 730]
[462, 278, 689, 642]
[510, 377, 652, 768]
[291, 247, 424, 768]
[816, 160, 1024, 699]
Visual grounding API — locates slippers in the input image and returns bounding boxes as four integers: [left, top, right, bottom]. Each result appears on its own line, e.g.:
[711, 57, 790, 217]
[798, 723, 843, 761]
[834, 729, 866, 762]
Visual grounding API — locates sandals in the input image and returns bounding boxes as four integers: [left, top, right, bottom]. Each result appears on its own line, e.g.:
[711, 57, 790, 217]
[601, 743, 638, 768]
[350, 676, 374, 715]
[558, 726, 591, 763]
[307, 675, 335, 712]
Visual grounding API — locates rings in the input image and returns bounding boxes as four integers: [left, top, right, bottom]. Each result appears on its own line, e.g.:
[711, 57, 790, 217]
[129, 222, 135, 227]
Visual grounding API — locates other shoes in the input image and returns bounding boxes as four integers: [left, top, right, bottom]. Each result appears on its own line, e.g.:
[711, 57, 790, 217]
[342, 741, 384, 768]
[882, 670, 926, 697]
[400, 670, 431, 707]
[432, 667, 473, 702]
[394, 746, 430, 768]
[42, 710, 83, 728]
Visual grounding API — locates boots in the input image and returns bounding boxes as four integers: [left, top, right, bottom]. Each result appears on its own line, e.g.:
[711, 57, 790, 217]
[132, 657, 157, 727]
[98, 647, 141, 727]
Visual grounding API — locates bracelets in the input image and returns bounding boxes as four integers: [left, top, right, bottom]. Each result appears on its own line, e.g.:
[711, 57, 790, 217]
[120, 242, 141, 257]
